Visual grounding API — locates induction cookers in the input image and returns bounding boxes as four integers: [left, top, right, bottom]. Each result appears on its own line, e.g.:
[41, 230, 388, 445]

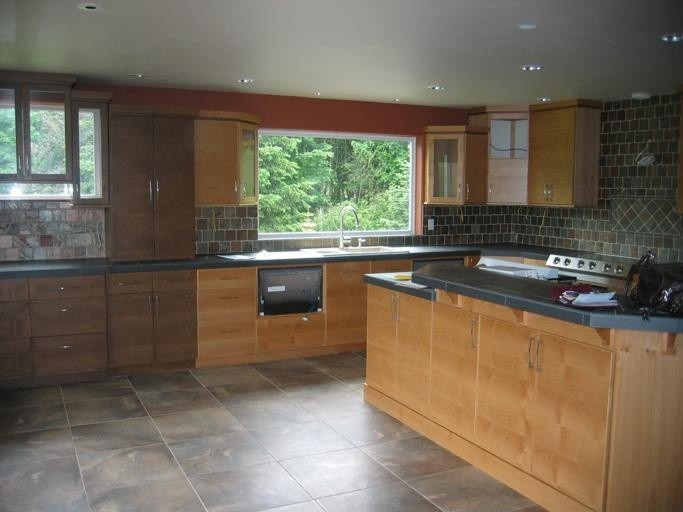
[551, 250, 660, 267]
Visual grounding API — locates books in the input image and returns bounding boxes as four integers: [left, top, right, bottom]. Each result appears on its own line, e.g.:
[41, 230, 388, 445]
[394, 280, 430, 290]
[570, 291, 618, 308]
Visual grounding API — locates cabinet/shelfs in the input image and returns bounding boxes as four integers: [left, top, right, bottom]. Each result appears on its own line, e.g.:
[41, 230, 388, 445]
[429, 273, 478, 465]
[424, 125, 489, 206]
[363, 272, 429, 437]
[109, 105, 196, 263]
[479, 290, 683, 511]
[370, 256, 547, 272]
[526, 99, 602, 208]
[194, 109, 259, 207]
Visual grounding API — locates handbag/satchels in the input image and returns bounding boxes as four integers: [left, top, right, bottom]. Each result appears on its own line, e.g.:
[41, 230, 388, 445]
[624, 251, 683, 321]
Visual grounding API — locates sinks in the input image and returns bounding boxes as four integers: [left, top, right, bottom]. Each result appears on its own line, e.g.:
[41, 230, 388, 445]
[300, 246, 410, 257]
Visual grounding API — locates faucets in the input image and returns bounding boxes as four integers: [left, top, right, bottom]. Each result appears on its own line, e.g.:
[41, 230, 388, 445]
[338, 205, 360, 249]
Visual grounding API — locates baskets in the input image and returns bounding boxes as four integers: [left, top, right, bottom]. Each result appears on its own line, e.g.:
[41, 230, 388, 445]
[550, 284, 592, 299]
